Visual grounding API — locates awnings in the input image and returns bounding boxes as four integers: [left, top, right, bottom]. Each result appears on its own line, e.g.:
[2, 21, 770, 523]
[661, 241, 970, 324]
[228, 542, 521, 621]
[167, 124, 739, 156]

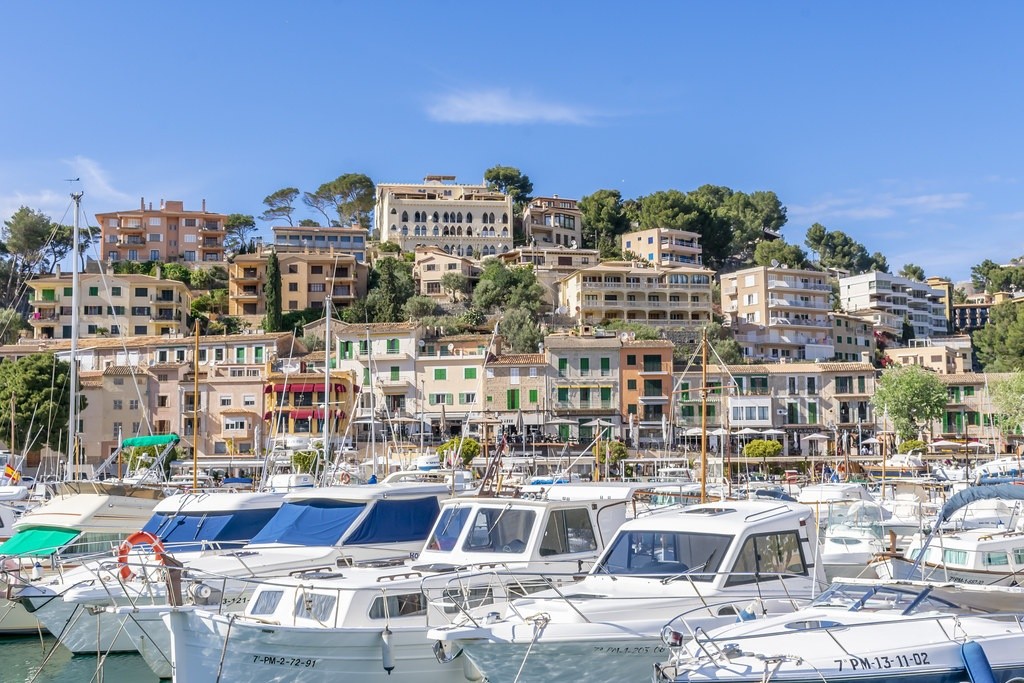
[291, 409, 346, 419]
[263, 384, 346, 394]
[263, 410, 272, 420]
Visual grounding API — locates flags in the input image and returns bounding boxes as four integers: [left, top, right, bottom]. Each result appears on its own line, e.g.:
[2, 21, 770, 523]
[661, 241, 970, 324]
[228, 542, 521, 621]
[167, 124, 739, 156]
[4, 463, 20, 483]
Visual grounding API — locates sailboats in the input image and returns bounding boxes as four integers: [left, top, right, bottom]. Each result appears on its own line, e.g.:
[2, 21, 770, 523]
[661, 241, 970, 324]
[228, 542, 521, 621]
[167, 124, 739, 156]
[2, 181, 1023, 682]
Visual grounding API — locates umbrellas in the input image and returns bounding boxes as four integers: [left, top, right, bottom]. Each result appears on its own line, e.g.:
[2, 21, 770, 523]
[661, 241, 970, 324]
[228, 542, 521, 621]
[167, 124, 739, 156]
[353, 412, 421, 440]
[581, 417, 615, 434]
[861, 437, 883, 448]
[895, 427, 991, 454]
[801, 433, 831, 449]
[662, 413, 668, 451]
[515, 407, 524, 434]
[629, 413, 634, 446]
[545, 418, 580, 435]
[439, 405, 446, 438]
[463, 412, 502, 442]
[676, 427, 787, 451]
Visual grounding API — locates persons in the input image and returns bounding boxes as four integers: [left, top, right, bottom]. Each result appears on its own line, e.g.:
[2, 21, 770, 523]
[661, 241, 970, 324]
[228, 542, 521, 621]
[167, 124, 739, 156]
[397, 426, 439, 442]
[861, 445, 868, 454]
[685, 442, 742, 455]
[503, 428, 559, 443]
[213, 467, 244, 486]
